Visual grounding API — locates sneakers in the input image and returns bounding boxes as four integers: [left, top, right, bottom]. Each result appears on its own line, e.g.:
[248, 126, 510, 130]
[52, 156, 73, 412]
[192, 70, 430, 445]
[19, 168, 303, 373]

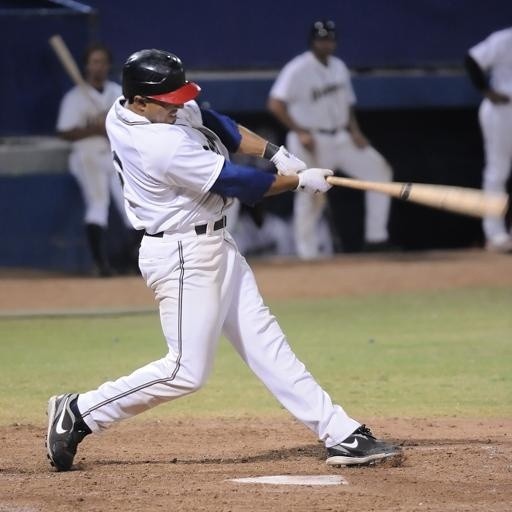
[325, 424, 405, 468]
[46, 392, 91, 471]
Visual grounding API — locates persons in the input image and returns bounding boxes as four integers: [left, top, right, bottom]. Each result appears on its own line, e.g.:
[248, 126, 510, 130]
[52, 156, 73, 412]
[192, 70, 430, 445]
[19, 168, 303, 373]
[233, 196, 287, 258]
[281, 214, 334, 258]
[267, 19, 397, 263]
[45, 47, 408, 472]
[463, 27, 511, 254]
[54, 43, 144, 279]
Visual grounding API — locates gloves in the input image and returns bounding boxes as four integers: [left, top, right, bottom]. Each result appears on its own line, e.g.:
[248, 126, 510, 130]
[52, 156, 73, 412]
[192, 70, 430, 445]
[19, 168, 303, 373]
[290, 167, 334, 194]
[268, 145, 307, 176]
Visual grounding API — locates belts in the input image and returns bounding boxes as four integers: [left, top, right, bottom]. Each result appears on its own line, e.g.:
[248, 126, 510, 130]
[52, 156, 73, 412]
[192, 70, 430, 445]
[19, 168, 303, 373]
[145, 215, 227, 238]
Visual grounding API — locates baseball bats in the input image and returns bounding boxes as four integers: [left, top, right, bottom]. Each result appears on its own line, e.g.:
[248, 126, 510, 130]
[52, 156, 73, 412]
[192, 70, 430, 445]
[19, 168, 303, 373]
[48, 32, 103, 113]
[325, 175, 509, 220]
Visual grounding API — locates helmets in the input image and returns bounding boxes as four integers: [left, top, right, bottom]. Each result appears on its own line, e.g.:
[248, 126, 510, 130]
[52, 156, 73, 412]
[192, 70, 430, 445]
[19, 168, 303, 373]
[122, 47, 202, 105]
[311, 20, 337, 38]
